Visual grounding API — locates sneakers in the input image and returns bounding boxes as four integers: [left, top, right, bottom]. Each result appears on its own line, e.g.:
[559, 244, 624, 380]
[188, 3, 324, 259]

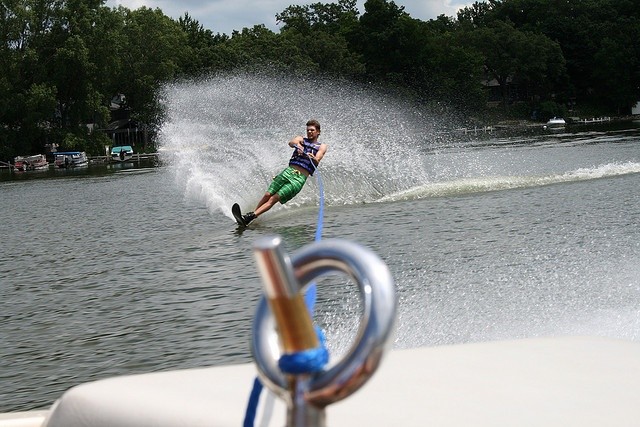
[241, 212, 256, 220]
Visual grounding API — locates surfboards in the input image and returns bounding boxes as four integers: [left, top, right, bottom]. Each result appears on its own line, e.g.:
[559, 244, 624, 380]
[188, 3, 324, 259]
[231, 202, 256, 231]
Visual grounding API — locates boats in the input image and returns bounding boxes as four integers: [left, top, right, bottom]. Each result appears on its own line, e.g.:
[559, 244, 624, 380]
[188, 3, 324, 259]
[53, 151, 87, 168]
[111, 146, 133, 162]
[14, 154, 49, 171]
[544, 117, 566, 127]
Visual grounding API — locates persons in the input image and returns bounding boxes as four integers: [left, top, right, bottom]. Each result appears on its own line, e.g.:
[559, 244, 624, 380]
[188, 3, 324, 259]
[231, 120, 325, 227]
